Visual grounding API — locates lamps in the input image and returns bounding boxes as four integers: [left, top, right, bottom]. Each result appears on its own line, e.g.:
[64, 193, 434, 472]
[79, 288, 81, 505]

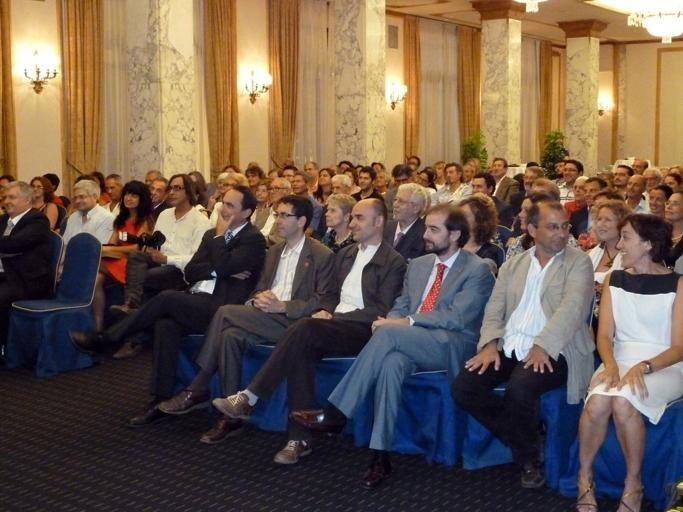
[237, 64, 275, 106]
[623, 0, 682, 45]
[597, 87, 615, 118]
[14, 43, 60, 94]
[384, 73, 410, 112]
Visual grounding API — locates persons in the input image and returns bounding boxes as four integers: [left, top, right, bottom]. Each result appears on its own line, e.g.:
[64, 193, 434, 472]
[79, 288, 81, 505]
[0, 157, 683, 511]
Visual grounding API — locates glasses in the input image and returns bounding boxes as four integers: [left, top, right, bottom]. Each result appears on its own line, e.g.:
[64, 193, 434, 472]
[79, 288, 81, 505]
[271, 212, 301, 220]
[70, 194, 92, 202]
[533, 222, 573, 234]
[266, 187, 289, 192]
[390, 196, 420, 208]
[167, 185, 184, 192]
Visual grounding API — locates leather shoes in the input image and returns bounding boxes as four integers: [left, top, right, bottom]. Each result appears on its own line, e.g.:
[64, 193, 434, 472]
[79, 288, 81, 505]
[69, 333, 120, 358]
[158, 390, 211, 415]
[126, 400, 173, 427]
[270, 438, 312, 466]
[114, 341, 143, 361]
[212, 390, 259, 420]
[290, 409, 345, 433]
[200, 417, 244, 444]
[358, 453, 391, 491]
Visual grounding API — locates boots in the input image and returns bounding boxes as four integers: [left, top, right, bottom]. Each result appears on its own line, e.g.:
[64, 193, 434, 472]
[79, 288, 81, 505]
[108, 297, 140, 318]
[517, 424, 553, 489]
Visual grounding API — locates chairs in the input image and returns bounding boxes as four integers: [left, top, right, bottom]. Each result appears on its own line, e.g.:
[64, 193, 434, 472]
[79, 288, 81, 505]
[386, 271, 513, 467]
[0, 232, 103, 376]
[154, 334, 224, 412]
[234, 339, 296, 443]
[466, 285, 596, 486]
[498, 223, 514, 249]
[293, 355, 378, 452]
[540, 386, 683, 512]
[49, 228, 64, 295]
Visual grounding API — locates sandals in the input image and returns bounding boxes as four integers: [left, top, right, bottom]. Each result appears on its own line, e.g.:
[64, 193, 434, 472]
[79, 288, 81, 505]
[572, 477, 600, 512]
[613, 487, 643, 512]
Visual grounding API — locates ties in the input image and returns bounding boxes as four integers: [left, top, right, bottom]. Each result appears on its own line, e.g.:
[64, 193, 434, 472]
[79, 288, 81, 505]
[224, 230, 233, 249]
[393, 231, 404, 250]
[419, 262, 449, 316]
[3, 220, 14, 240]
